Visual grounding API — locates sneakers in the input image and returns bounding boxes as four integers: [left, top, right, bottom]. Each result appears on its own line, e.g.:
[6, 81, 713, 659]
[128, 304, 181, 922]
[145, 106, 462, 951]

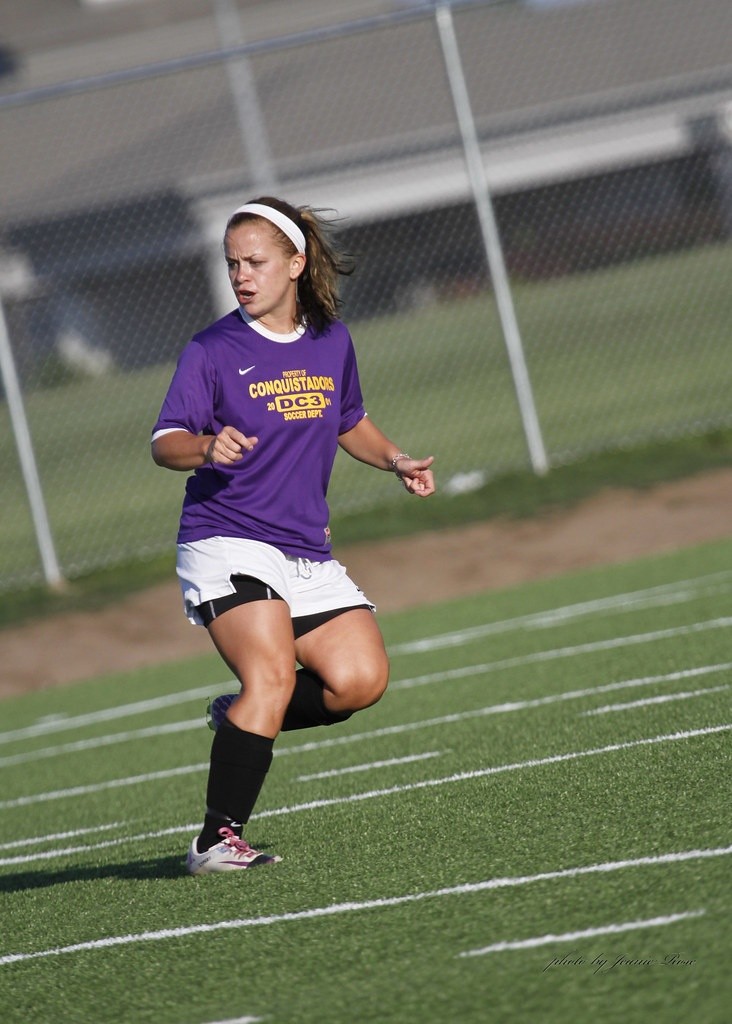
[207, 694, 238, 730]
[187, 827, 285, 876]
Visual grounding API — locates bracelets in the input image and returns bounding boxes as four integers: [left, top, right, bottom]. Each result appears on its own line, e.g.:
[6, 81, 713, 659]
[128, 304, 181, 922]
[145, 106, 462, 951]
[392, 453, 412, 481]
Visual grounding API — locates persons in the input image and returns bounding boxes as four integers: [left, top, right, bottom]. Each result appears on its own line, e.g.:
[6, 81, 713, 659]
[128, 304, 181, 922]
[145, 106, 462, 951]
[150, 195, 435, 875]
